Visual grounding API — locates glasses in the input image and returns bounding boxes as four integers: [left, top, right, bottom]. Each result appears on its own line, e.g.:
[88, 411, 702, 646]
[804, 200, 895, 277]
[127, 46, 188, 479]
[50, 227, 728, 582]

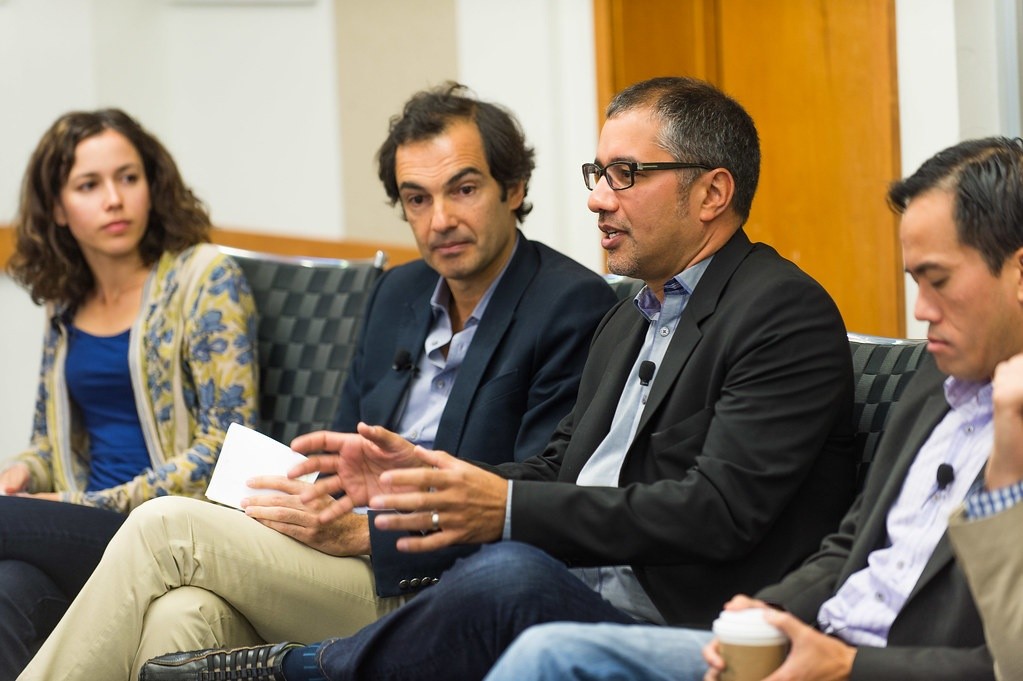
[582, 160, 711, 191]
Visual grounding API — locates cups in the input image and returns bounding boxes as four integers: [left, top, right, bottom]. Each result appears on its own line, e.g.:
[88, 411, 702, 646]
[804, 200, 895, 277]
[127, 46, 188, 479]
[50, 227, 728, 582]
[713, 609, 790, 681]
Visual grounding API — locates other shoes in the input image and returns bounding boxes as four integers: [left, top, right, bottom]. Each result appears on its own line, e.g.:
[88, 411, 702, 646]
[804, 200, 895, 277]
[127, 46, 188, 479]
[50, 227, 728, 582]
[138, 640, 307, 681]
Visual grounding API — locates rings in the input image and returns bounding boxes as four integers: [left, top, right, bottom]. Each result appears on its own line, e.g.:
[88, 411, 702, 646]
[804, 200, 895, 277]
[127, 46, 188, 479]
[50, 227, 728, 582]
[432, 510, 438, 531]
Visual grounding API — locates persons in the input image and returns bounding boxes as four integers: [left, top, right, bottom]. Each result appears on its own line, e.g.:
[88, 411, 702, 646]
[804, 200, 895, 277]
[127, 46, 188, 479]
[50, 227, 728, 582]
[0, 108, 264, 681]
[138, 75, 860, 681]
[482, 130, 1023, 681]
[943, 350, 1023, 681]
[14, 79, 617, 681]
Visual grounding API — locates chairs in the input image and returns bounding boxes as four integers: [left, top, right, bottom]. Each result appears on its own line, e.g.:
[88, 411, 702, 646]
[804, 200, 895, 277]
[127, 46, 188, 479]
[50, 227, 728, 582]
[207, 243, 930, 497]
